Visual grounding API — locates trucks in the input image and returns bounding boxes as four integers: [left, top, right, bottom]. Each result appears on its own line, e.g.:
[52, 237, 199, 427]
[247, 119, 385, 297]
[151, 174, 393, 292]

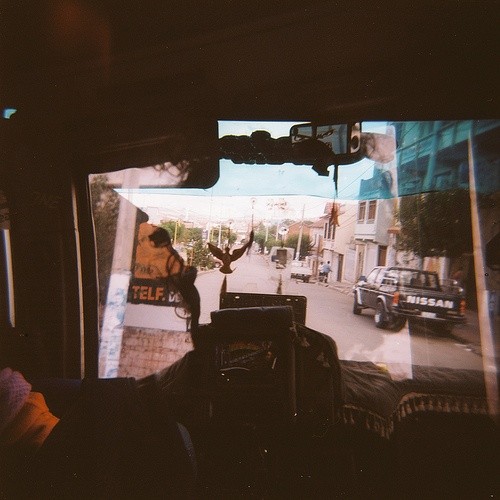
[290, 260, 312, 283]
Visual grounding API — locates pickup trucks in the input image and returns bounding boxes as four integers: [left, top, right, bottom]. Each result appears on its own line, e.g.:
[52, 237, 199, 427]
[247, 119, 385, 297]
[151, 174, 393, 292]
[353, 266, 468, 335]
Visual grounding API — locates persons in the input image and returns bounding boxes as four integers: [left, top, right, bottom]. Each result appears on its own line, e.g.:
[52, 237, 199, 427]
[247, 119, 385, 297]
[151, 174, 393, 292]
[321, 261, 333, 283]
[0, 322, 83, 500]
[453, 267, 468, 288]
[318, 262, 325, 282]
